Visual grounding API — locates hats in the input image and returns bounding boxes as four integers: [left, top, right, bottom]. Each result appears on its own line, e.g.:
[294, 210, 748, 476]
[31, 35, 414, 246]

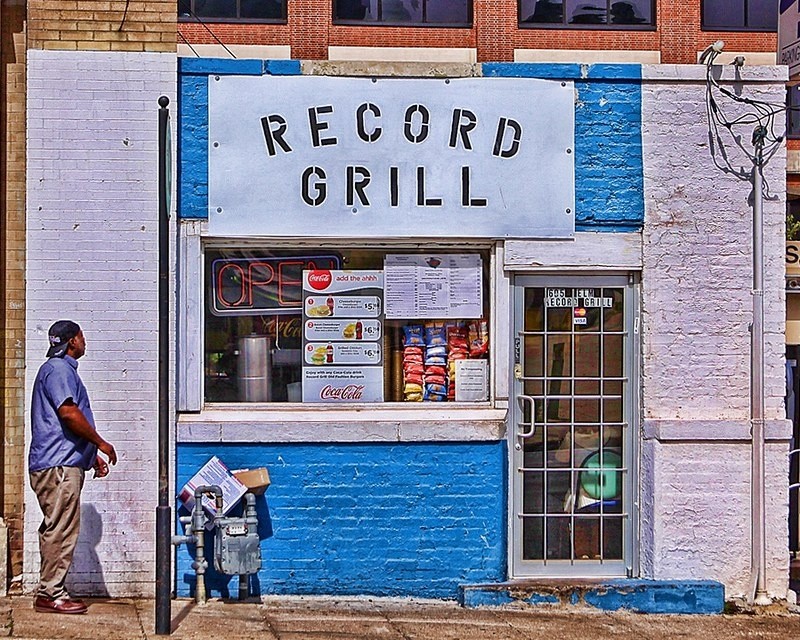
[45, 320, 80, 357]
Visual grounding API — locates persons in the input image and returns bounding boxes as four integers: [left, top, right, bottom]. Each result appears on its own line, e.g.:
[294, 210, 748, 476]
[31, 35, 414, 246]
[26, 320, 120, 615]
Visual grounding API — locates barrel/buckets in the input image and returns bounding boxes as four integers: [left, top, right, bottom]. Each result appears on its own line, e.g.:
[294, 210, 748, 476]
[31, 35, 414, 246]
[233, 331, 273, 402]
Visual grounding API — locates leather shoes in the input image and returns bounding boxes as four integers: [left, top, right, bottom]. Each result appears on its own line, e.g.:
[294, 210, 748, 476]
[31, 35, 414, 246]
[35, 595, 88, 613]
